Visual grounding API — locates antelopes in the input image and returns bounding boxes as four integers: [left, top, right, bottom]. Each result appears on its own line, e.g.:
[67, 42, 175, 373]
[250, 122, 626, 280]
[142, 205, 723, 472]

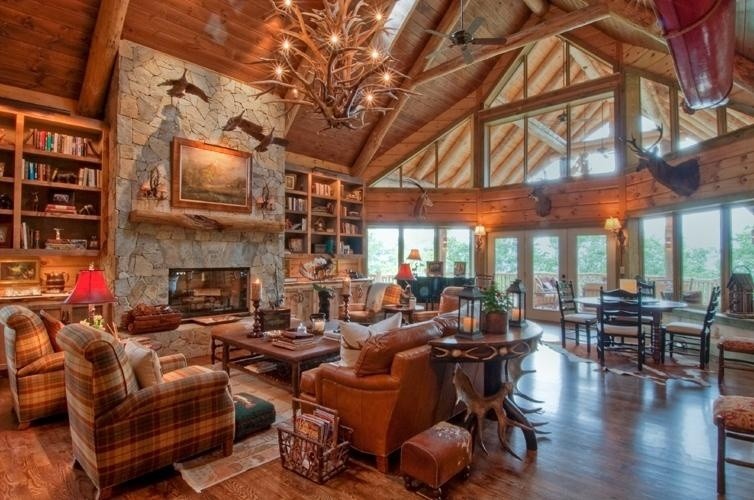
[402, 176, 434, 222]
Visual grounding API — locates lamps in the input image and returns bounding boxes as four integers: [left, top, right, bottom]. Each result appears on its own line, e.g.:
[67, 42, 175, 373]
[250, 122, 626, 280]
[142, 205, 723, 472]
[406, 249, 422, 260]
[505, 278, 529, 327]
[473, 224, 485, 248]
[253, 1, 422, 129]
[393, 264, 417, 283]
[605, 215, 627, 248]
[63, 269, 118, 327]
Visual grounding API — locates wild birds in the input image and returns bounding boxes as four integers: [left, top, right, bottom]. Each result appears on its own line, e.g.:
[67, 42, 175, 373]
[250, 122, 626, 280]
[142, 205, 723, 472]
[254, 127, 288, 153]
[156, 68, 212, 103]
[221, 109, 263, 137]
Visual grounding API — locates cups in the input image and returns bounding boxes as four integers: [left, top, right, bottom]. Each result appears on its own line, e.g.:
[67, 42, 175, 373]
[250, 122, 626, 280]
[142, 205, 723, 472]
[408, 297, 416, 309]
[297, 325, 308, 334]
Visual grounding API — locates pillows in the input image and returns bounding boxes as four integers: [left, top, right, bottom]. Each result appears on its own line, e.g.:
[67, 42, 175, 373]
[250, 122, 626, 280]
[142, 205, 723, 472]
[125, 337, 164, 388]
[40, 308, 64, 353]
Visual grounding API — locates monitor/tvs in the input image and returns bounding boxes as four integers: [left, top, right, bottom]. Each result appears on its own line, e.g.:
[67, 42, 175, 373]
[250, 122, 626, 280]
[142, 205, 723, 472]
[168, 267, 248, 318]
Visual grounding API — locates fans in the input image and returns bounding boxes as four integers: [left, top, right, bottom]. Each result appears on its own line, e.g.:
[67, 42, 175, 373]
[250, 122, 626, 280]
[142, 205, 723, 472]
[418, 0, 506, 65]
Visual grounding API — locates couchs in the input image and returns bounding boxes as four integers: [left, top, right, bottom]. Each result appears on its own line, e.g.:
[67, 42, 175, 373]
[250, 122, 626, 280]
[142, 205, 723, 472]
[300, 279, 513, 474]
[56, 324, 236, 499]
[1, 304, 69, 431]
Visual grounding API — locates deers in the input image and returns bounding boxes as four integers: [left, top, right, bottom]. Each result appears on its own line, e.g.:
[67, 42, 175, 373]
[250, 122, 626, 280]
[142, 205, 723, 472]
[522, 177, 552, 218]
[618, 122, 699, 196]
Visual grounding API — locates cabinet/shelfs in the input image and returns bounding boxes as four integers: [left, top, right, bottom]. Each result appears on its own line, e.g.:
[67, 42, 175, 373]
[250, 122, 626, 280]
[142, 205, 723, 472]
[0, 107, 107, 259]
[284, 169, 368, 258]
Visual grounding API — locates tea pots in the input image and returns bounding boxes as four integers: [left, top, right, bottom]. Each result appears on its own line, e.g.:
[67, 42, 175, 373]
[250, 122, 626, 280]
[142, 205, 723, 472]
[42, 268, 71, 294]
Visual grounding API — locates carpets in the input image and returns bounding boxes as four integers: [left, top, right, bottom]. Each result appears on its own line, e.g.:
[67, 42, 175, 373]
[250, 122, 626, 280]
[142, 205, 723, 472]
[543, 337, 712, 390]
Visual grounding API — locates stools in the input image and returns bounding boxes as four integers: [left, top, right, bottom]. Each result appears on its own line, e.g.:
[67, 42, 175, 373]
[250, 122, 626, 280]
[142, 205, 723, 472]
[716, 333, 754, 385]
[712, 394, 754, 500]
[400, 421, 472, 500]
[232, 392, 276, 444]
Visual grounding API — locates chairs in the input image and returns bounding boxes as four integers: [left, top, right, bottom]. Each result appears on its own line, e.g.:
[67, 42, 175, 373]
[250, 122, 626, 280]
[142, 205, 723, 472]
[552, 279, 597, 354]
[620, 275, 655, 350]
[597, 284, 646, 371]
[661, 285, 720, 368]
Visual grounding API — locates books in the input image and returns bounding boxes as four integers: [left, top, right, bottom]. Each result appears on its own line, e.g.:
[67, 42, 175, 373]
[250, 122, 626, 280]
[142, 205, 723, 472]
[292, 403, 338, 467]
[286, 170, 362, 255]
[21, 123, 103, 250]
[244, 361, 277, 374]
[273, 331, 316, 351]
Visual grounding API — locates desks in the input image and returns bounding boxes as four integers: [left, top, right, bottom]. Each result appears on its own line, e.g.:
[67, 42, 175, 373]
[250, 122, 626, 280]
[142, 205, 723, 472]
[398, 277, 475, 312]
[429, 318, 543, 451]
[570, 294, 687, 361]
[210, 319, 341, 408]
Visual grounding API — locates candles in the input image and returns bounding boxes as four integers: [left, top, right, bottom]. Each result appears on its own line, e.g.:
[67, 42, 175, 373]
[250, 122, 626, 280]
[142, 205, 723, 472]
[251, 279, 262, 302]
[341, 279, 352, 296]
[315, 320, 325, 332]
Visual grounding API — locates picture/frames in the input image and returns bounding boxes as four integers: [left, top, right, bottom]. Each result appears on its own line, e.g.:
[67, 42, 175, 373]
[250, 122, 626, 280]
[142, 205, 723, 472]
[170, 136, 256, 215]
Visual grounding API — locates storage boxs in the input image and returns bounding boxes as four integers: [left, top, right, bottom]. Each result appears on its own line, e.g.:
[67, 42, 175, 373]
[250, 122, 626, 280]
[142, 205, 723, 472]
[277, 423, 354, 485]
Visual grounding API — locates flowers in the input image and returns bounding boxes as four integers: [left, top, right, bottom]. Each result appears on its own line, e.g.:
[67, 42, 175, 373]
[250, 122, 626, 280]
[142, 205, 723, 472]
[303, 253, 333, 294]
